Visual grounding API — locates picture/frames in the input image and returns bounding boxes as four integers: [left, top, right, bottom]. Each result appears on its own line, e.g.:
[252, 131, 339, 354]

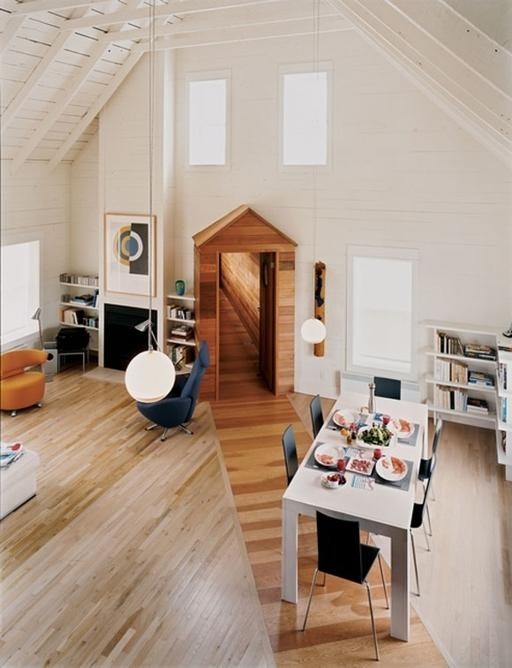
[102, 211, 157, 298]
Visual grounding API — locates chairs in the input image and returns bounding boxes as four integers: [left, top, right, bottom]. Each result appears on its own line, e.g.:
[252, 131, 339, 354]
[421, 417, 444, 536]
[301, 508, 390, 662]
[135, 339, 211, 441]
[373, 375, 402, 399]
[280, 422, 299, 485]
[364, 454, 435, 608]
[310, 393, 325, 439]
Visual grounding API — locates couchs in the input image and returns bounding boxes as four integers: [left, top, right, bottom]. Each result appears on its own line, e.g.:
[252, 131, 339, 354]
[0, 346, 49, 417]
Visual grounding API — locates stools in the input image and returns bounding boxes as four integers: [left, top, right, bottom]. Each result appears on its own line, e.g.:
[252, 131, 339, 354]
[57, 348, 89, 374]
[0, 441, 41, 520]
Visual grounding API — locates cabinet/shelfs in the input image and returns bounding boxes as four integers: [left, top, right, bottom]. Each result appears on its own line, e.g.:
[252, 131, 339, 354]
[164, 292, 196, 369]
[421, 319, 498, 430]
[56, 279, 98, 352]
[494, 334, 512, 481]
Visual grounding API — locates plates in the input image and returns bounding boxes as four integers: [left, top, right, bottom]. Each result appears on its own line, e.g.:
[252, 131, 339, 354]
[332, 407, 361, 427]
[320, 470, 349, 488]
[313, 441, 347, 466]
[375, 454, 409, 482]
[386, 414, 416, 438]
[345, 456, 375, 475]
[356, 424, 397, 449]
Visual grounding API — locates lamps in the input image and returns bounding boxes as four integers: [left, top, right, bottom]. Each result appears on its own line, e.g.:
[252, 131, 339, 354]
[29, 307, 44, 348]
[299, 317, 328, 345]
[124, 0, 177, 404]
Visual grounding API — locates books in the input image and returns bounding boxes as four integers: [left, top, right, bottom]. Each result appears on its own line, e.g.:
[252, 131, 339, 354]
[0, 443, 23, 471]
[495, 353, 507, 453]
[435, 332, 496, 417]
[58, 268, 99, 329]
[164, 301, 193, 369]
